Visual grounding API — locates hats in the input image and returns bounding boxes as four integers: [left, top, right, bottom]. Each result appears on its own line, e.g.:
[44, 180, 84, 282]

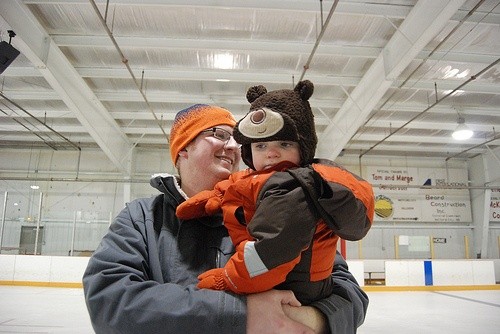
[233, 80, 318, 171]
[169, 104, 237, 167]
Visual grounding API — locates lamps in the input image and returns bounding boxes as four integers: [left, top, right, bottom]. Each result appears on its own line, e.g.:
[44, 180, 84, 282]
[0, 29, 20, 74]
[451, 117, 473, 140]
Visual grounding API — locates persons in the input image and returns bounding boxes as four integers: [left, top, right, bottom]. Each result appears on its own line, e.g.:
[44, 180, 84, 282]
[81, 104, 369, 334]
[175, 80, 374, 306]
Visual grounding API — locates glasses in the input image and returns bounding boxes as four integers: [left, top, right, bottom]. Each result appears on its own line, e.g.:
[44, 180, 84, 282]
[200, 127, 242, 149]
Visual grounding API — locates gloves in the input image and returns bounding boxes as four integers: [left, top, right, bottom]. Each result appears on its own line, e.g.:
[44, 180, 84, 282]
[197, 268, 231, 292]
[175, 180, 234, 219]
